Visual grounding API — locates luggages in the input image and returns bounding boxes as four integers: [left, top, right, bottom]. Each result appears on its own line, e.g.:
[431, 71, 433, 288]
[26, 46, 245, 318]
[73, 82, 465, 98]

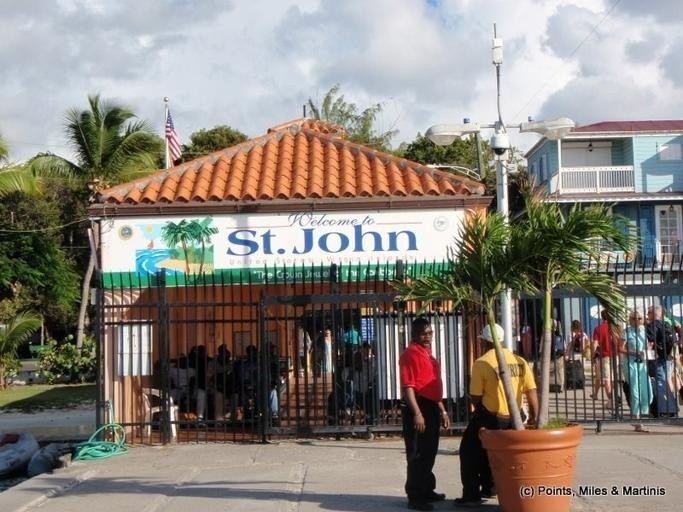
[565, 360, 585, 389]
[651, 379, 679, 418]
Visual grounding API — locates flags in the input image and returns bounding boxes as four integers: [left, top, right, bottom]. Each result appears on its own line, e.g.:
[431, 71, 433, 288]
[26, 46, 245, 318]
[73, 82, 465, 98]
[165, 111, 182, 162]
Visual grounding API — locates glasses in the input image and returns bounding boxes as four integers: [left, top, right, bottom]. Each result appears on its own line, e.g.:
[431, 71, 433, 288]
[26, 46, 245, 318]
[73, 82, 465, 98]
[418, 330, 434, 336]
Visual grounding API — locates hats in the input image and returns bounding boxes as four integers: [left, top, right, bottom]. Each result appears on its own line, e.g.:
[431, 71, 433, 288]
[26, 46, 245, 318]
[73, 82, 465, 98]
[477, 323, 505, 344]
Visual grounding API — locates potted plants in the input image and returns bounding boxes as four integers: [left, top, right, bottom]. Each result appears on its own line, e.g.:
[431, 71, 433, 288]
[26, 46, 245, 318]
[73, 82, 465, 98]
[384, 162, 644, 512]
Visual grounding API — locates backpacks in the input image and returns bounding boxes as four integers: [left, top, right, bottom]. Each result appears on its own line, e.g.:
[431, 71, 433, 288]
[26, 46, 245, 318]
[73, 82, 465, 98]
[573, 335, 587, 353]
[652, 320, 674, 358]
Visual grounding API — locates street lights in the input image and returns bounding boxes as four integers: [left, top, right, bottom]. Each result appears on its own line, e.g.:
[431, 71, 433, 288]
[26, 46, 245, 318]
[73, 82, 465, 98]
[425, 119, 574, 352]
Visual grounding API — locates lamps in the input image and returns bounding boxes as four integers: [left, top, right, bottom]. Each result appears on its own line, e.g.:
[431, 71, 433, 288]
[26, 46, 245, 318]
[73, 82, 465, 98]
[588, 139, 593, 152]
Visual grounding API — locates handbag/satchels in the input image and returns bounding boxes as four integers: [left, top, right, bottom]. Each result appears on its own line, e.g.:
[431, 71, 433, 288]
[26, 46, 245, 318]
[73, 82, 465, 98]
[552, 334, 565, 362]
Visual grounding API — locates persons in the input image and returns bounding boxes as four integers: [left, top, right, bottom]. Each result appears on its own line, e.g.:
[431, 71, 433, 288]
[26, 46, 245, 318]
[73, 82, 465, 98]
[398, 317, 451, 512]
[454, 321, 539, 507]
[519, 304, 682, 433]
[166, 322, 376, 428]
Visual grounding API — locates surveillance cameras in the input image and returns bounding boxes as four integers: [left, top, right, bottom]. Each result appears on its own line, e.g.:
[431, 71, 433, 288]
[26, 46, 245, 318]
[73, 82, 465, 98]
[491, 134, 510, 155]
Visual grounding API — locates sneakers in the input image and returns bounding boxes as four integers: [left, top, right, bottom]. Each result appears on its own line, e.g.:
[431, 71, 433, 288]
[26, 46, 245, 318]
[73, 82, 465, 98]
[454, 496, 481, 507]
[408, 499, 434, 511]
[429, 494, 445, 501]
[635, 425, 650, 432]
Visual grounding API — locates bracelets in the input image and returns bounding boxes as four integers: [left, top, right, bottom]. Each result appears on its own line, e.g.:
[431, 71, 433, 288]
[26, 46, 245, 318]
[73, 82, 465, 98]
[412, 411, 421, 418]
[439, 411, 447, 415]
